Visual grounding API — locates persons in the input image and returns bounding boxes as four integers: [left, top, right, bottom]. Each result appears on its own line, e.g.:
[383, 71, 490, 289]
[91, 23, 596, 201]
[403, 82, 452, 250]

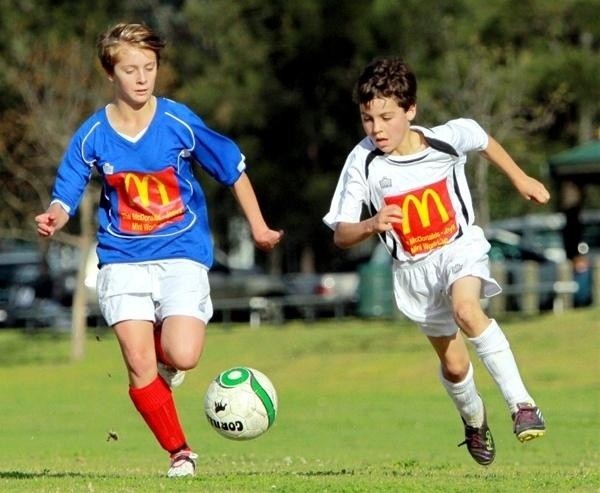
[32, 17, 284, 478]
[321, 56, 551, 464]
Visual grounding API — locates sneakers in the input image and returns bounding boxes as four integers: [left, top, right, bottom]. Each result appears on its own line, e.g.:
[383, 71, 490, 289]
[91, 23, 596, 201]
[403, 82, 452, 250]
[166, 450, 198, 478]
[158, 362, 185, 388]
[512, 402, 546, 442]
[458, 394, 496, 465]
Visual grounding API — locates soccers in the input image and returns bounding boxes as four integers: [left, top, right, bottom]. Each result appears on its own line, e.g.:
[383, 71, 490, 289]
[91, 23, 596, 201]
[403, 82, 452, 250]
[204, 366, 277, 440]
